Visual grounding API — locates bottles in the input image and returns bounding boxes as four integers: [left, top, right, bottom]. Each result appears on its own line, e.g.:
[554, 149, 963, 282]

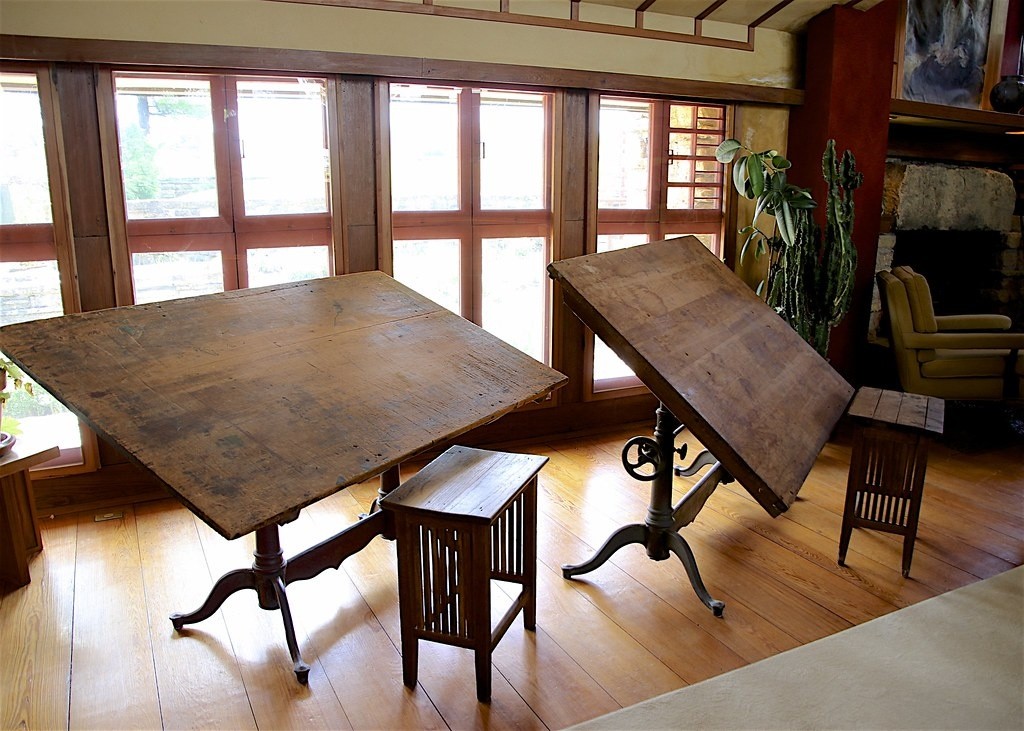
[989, 75, 1024, 114]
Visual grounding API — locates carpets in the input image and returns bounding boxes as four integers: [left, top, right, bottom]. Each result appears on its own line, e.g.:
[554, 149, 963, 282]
[563, 565, 1024, 731]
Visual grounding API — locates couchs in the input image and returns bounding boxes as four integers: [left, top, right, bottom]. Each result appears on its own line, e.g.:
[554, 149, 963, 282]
[875, 266, 1023, 441]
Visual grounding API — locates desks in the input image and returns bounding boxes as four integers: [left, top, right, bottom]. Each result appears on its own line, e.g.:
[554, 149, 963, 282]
[0, 274, 567, 690]
[0, 440, 61, 590]
[549, 235, 854, 616]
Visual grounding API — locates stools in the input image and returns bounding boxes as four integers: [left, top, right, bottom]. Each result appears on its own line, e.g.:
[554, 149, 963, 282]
[378, 446, 550, 703]
[839, 387, 944, 577]
